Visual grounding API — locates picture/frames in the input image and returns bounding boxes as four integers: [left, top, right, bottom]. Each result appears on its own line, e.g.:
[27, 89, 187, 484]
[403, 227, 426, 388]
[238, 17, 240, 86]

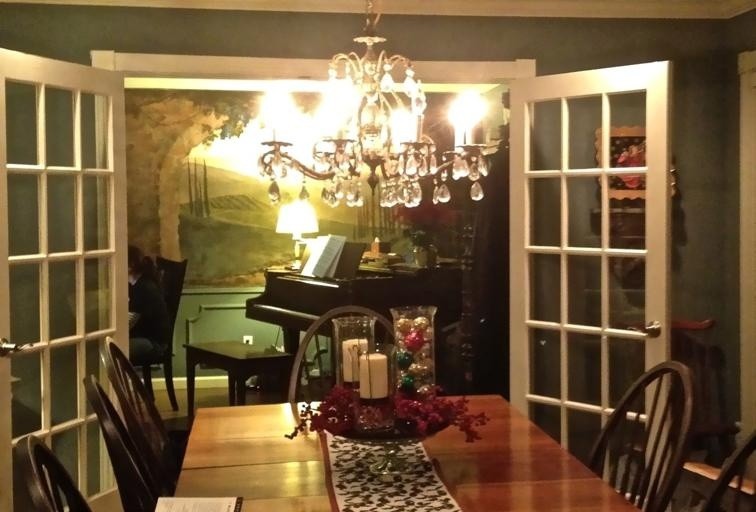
[595, 125, 676, 200]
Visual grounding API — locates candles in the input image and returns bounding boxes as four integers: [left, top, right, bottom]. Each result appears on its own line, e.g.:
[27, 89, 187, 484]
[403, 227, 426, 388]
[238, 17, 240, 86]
[359, 353, 388, 399]
[342, 339, 368, 382]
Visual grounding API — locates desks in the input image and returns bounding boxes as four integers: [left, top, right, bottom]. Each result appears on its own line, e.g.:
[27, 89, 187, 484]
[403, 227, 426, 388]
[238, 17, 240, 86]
[174, 394, 644, 512]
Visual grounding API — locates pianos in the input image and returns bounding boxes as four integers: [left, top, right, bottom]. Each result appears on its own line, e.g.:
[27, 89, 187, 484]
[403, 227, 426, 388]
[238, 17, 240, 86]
[245, 269, 463, 387]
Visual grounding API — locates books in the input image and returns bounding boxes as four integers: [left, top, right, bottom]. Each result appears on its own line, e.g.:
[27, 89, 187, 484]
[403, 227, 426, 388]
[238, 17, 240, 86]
[154, 497, 243, 512]
[300, 234, 345, 277]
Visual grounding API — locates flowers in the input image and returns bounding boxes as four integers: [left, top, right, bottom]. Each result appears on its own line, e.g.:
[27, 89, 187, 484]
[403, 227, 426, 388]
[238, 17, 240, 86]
[285, 386, 490, 443]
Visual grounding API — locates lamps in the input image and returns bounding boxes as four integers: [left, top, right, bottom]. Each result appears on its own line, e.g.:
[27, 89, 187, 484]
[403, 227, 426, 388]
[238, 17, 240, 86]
[257, 0, 500, 209]
[275, 203, 319, 271]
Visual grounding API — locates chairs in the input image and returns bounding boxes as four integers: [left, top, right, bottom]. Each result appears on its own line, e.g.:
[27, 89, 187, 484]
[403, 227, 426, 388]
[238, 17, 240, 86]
[699, 429, 756, 512]
[83, 373, 162, 512]
[133, 255, 189, 412]
[13, 436, 93, 512]
[101, 336, 190, 497]
[588, 361, 702, 512]
[287, 304, 394, 402]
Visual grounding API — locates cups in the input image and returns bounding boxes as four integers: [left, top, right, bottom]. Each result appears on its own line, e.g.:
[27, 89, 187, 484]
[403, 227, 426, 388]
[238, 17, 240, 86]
[388, 307, 438, 402]
[357, 343, 398, 402]
[331, 314, 376, 383]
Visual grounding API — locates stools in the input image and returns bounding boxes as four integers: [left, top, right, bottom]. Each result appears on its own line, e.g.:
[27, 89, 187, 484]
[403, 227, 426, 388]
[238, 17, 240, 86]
[182, 341, 296, 417]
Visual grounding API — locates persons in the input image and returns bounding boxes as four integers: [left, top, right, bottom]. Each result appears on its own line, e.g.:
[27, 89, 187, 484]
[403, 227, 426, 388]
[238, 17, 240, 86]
[127, 243, 166, 365]
